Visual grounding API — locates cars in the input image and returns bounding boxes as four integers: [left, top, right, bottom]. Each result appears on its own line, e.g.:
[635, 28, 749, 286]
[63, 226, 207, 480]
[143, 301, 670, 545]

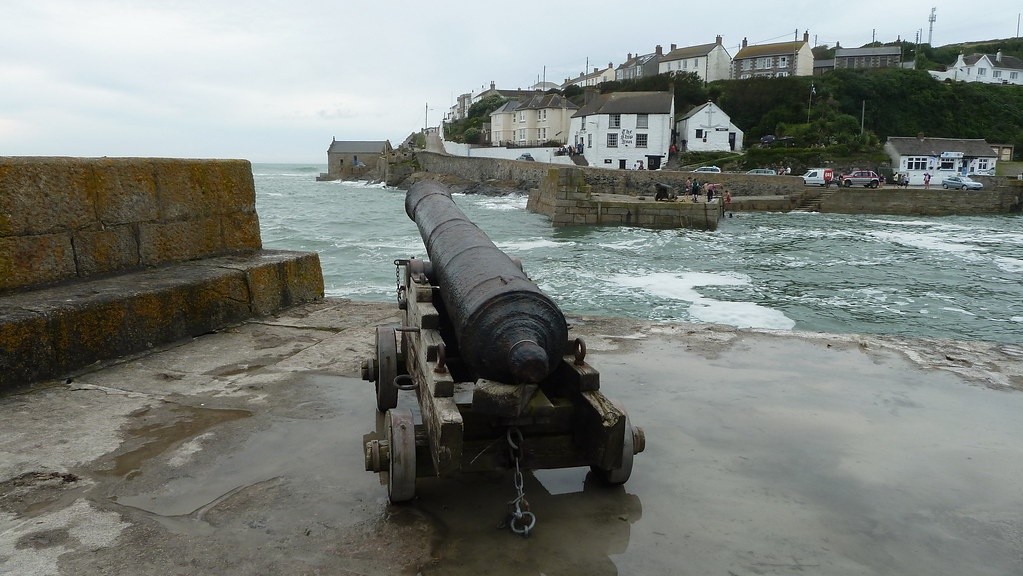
[941, 176, 984, 191]
[515, 153, 534, 161]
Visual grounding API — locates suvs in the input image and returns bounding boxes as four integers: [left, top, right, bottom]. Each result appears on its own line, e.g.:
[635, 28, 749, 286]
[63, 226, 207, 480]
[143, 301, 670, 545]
[842, 171, 881, 188]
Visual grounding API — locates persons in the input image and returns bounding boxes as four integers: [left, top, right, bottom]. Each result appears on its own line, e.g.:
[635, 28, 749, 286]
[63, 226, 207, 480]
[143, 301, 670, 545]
[780, 166, 791, 175]
[893, 172, 909, 189]
[632, 161, 644, 170]
[839, 173, 846, 189]
[685, 177, 731, 210]
[924, 173, 930, 189]
[560, 144, 584, 156]
[879, 173, 885, 188]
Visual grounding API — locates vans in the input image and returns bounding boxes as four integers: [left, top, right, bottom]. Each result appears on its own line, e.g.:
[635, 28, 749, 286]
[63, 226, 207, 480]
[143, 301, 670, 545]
[798, 168, 834, 185]
[745, 169, 777, 175]
[690, 166, 721, 173]
